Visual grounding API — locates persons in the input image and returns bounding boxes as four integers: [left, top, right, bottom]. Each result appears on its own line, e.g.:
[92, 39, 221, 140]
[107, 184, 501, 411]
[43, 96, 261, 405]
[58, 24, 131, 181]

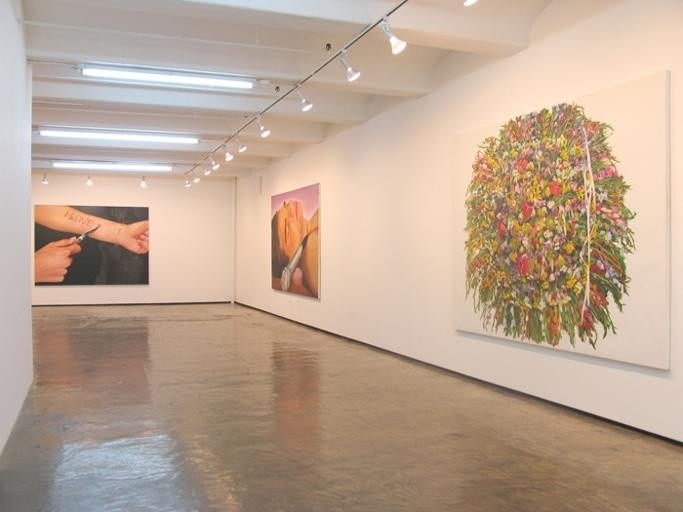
[35, 205, 148, 283]
[272, 200, 319, 297]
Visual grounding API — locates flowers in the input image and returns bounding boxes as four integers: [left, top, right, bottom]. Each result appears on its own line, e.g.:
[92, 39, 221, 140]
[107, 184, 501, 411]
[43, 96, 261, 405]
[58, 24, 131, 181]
[463, 103, 637, 348]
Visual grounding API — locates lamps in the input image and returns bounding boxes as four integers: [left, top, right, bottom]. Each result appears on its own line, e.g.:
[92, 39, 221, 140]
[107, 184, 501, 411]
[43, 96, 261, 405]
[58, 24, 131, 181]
[40, 172, 49, 185]
[49, 161, 173, 173]
[140, 176, 147, 188]
[70, 65, 255, 91]
[33, 126, 201, 145]
[182, 0, 477, 188]
[86, 176, 93, 185]
